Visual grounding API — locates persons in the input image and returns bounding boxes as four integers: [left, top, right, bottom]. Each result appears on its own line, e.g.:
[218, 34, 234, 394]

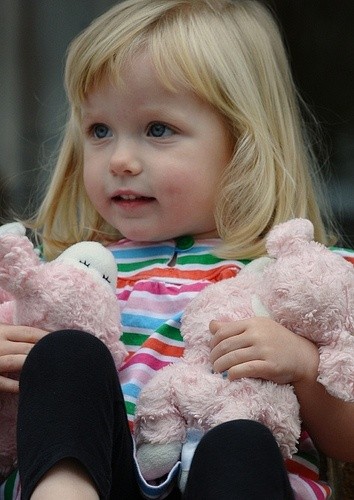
[0, 0, 354, 500]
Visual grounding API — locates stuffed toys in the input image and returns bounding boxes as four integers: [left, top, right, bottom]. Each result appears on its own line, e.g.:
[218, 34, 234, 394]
[135, 217, 353, 482]
[1, 222, 128, 485]
